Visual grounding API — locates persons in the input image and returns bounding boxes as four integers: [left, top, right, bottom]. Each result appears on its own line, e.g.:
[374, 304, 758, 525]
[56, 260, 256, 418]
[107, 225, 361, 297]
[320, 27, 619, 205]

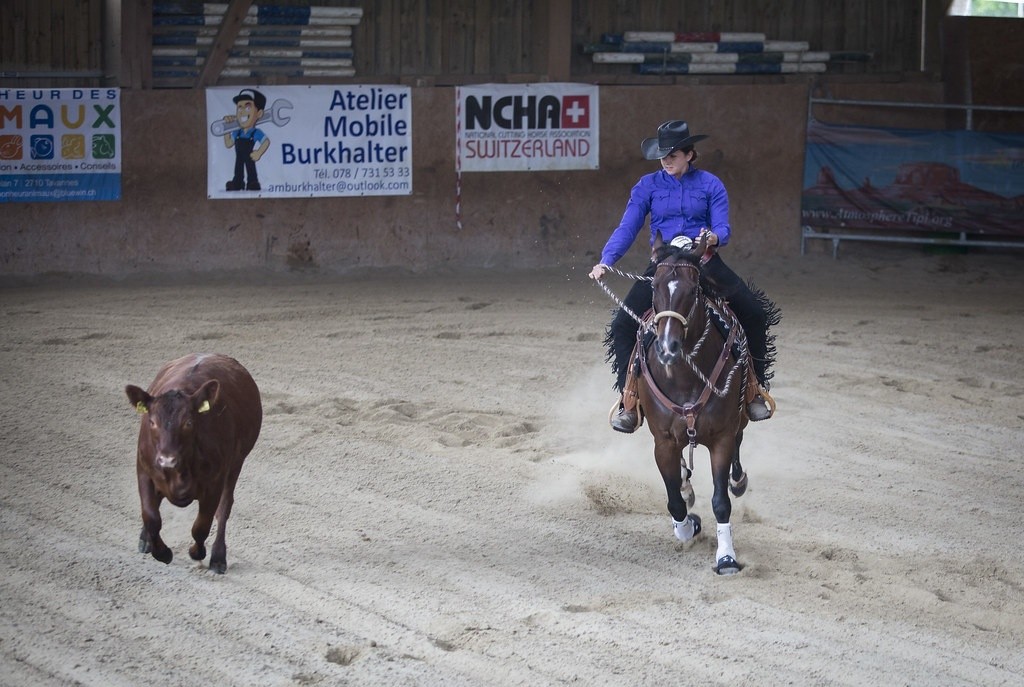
[589, 120, 776, 434]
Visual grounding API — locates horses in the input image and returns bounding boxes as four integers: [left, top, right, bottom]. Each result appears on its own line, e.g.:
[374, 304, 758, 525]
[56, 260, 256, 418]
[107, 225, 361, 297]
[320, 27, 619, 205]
[636, 227, 751, 576]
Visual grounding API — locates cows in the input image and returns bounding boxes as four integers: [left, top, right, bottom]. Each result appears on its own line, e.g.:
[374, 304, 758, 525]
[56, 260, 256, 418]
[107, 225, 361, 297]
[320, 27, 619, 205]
[125, 351, 263, 576]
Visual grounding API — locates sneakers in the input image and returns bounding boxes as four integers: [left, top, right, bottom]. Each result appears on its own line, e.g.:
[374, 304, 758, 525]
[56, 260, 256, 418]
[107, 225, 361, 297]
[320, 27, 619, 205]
[746, 394, 770, 421]
[612, 408, 643, 433]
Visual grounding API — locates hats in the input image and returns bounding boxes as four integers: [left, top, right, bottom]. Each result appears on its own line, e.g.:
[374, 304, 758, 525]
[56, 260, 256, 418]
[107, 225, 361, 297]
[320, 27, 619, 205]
[641, 120, 711, 160]
